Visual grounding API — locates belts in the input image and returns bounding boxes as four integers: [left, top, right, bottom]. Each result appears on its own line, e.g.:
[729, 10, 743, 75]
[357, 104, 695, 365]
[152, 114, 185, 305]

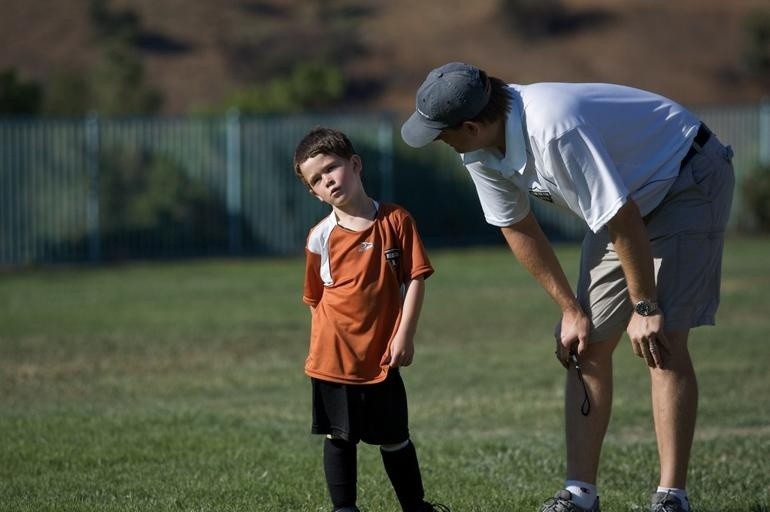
[679, 121, 712, 167]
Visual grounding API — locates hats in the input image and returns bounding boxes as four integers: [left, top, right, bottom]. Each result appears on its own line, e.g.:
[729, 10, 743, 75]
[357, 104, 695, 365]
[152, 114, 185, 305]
[400, 61, 492, 148]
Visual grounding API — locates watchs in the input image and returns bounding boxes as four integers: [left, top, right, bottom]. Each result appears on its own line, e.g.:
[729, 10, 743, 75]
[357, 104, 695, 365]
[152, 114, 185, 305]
[633, 300, 659, 316]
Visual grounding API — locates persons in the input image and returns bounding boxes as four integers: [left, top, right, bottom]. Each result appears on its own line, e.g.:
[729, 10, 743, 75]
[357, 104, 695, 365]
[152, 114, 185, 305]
[294, 128, 452, 512]
[401, 62, 735, 512]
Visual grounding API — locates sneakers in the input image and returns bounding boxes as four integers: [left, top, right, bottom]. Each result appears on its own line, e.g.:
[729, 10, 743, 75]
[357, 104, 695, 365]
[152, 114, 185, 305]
[650, 492, 690, 512]
[540, 489, 599, 512]
